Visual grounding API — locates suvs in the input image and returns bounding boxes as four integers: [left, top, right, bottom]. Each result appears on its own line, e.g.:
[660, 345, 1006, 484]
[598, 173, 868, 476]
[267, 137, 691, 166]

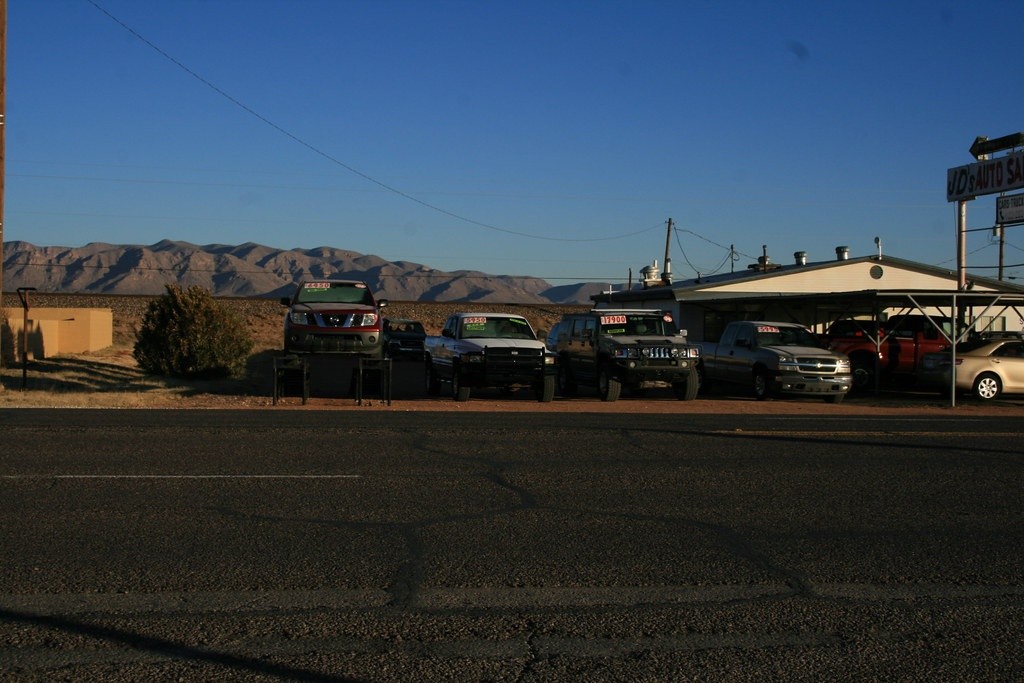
[382, 318, 430, 359]
[284, 276, 388, 354]
[550, 308, 706, 403]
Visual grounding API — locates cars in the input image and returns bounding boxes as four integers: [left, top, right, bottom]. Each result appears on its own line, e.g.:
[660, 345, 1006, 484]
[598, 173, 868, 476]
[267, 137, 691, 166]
[923, 338, 1024, 402]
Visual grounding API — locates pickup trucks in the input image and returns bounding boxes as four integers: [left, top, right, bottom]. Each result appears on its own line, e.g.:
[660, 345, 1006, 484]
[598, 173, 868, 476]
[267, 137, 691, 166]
[692, 318, 854, 404]
[422, 313, 560, 404]
[825, 314, 986, 394]
